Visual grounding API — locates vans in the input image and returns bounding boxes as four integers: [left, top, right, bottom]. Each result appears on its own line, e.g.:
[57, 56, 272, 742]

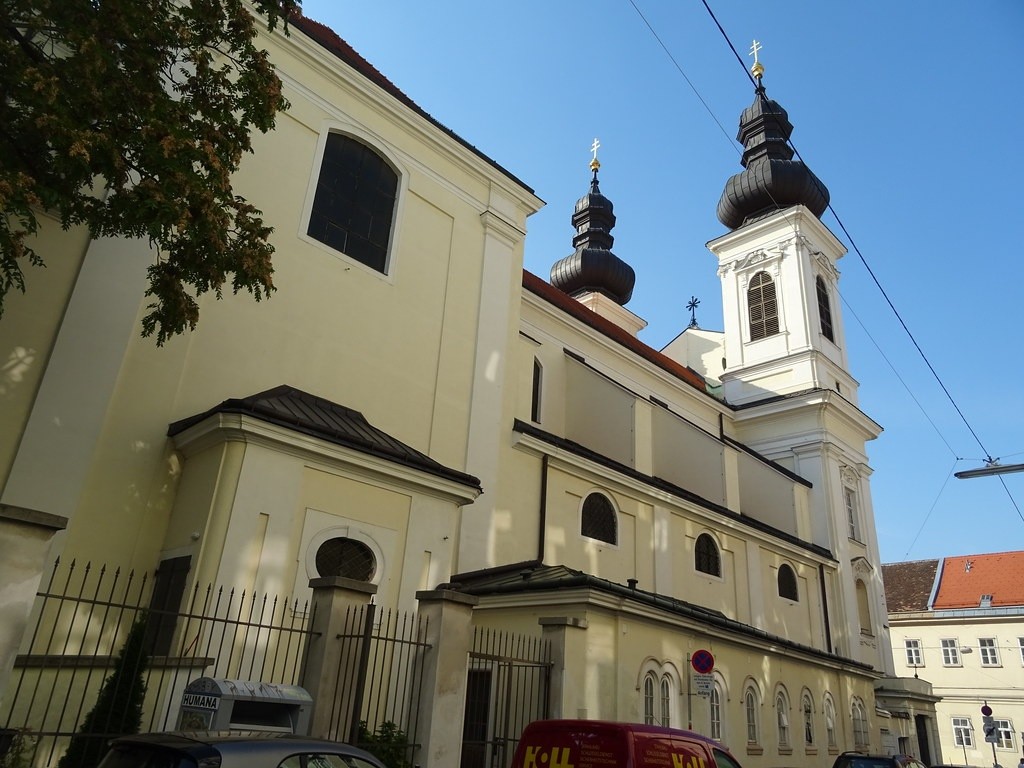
[510, 717, 746, 768]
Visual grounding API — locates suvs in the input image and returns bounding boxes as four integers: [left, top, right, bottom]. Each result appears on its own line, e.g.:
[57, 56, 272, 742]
[832, 750, 927, 768]
[96, 730, 388, 768]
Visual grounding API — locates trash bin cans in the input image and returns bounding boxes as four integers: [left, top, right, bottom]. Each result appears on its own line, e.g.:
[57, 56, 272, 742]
[172, 676, 315, 735]
